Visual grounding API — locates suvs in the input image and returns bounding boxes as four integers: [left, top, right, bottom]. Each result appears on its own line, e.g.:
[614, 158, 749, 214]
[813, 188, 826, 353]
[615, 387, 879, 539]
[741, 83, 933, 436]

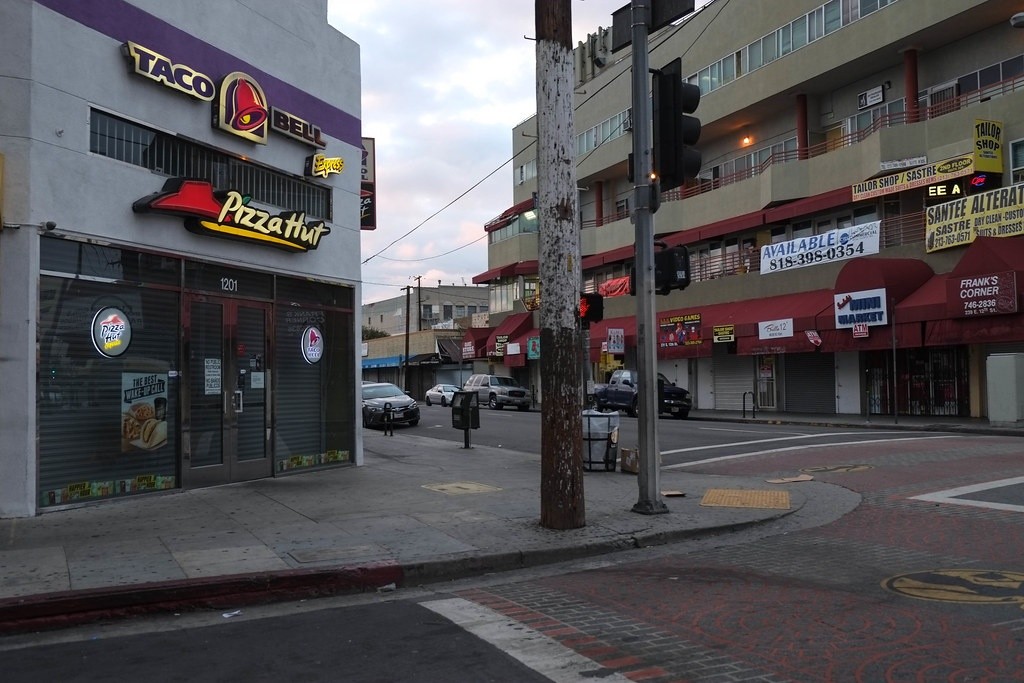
[464, 374, 531, 412]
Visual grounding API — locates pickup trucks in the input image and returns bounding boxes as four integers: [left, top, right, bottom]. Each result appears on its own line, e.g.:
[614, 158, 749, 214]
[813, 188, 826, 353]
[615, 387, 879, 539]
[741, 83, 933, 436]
[594, 370, 693, 420]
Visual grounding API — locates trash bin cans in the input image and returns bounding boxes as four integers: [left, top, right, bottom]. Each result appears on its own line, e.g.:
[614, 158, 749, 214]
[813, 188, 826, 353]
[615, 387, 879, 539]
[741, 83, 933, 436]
[582, 414, 619, 472]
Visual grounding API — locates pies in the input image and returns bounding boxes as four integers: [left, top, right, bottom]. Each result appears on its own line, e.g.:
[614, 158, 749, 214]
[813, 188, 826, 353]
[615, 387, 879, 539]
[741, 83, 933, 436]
[122, 402, 167, 448]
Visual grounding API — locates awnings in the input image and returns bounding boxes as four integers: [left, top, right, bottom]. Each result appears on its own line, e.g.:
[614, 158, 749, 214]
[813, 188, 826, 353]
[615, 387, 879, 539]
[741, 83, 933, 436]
[438, 312, 540, 367]
[361, 353, 474, 369]
[473, 260, 539, 284]
[589, 288, 835, 363]
[654, 185, 878, 253]
[581, 245, 635, 271]
[816, 236, 1024, 353]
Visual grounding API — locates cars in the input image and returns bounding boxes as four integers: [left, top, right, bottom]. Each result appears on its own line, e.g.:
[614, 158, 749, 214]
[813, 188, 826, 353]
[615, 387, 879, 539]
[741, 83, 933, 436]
[361, 380, 421, 428]
[425, 383, 463, 407]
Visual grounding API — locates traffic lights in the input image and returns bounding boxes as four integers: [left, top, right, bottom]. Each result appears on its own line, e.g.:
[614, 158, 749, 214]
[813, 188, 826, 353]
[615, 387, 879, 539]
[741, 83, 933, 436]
[579, 291, 604, 330]
[654, 59, 705, 188]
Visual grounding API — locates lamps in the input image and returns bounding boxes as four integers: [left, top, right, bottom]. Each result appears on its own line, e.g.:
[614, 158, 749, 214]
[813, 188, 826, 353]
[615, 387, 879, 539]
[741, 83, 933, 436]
[743, 133, 749, 144]
[884, 81, 891, 89]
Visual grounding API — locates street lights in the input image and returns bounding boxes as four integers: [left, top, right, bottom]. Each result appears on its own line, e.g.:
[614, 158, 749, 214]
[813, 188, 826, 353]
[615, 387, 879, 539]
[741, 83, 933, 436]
[400, 286, 413, 397]
